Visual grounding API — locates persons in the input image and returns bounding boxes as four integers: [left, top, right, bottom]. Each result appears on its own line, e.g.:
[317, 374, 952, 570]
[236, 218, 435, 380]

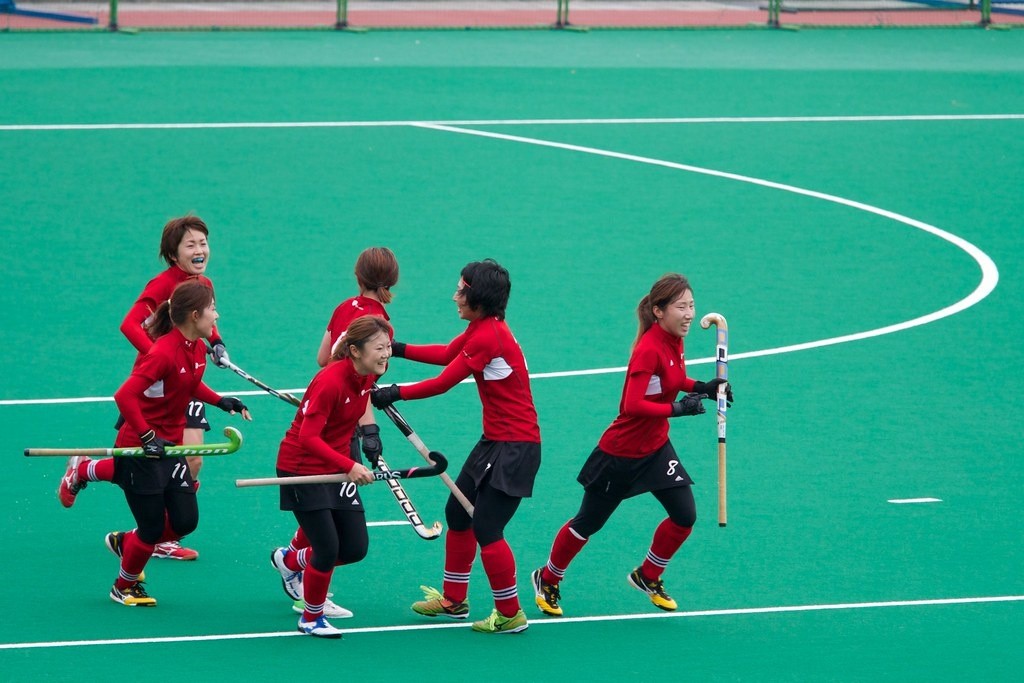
[59, 214, 230, 560]
[104, 281, 252, 607]
[369, 258, 542, 634]
[267, 317, 395, 638]
[530, 272, 733, 619]
[271, 245, 398, 620]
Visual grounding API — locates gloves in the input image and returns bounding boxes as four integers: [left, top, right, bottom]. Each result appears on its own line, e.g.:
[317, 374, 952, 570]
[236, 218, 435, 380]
[210, 342, 229, 369]
[370, 384, 403, 410]
[139, 429, 176, 460]
[361, 424, 382, 469]
[391, 339, 406, 358]
[692, 378, 734, 408]
[670, 392, 709, 417]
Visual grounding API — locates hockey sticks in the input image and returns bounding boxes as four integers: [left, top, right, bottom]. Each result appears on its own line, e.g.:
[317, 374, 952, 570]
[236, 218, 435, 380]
[204, 344, 304, 408]
[372, 380, 476, 519]
[22, 426, 245, 459]
[698, 310, 729, 529]
[234, 449, 450, 490]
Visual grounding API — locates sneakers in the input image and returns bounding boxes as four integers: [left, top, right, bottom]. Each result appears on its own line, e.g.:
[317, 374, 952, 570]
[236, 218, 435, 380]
[530, 566, 563, 616]
[297, 615, 342, 638]
[105, 531, 145, 582]
[108, 582, 156, 606]
[626, 565, 678, 611]
[291, 592, 354, 619]
[410, 591, 469, 619]
[271, 547, 303, 601]
[150, 542, 199, 560]
[471, 609, 529, 633]
[57, 454, 91, 508]
